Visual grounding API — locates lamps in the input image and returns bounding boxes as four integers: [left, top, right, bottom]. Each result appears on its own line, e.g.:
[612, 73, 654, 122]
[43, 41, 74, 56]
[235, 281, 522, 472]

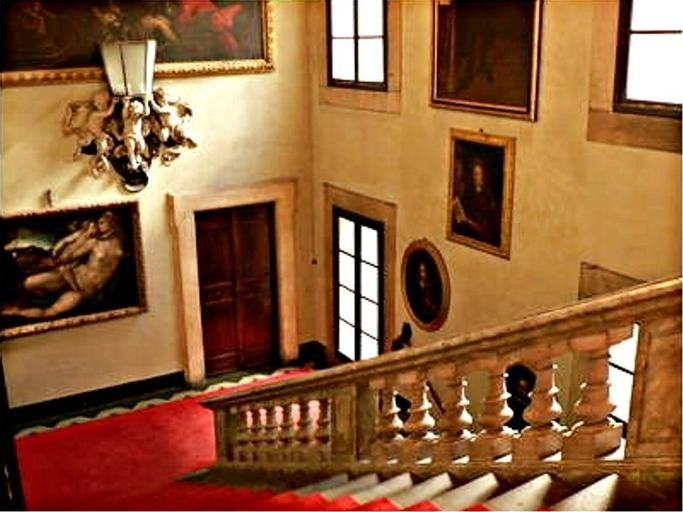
[58, 30, 198, 196]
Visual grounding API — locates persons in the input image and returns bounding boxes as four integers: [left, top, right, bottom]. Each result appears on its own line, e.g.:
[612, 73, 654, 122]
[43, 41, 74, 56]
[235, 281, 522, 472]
[3, 209, 123, 318]
[62, 84, 200, 179]
[452, 157, 501, 247]
[418, 264, 437, 315]
[34, 219, 98, 292]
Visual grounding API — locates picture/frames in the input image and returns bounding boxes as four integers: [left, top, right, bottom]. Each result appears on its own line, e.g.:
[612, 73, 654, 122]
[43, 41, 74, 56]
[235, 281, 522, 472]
[443, 125, 517, 261]
[0, 199, 149, 341]
[428, 0, 546, 122]
[0, 0, 279, 87]
[398, 237, 453, 332]
